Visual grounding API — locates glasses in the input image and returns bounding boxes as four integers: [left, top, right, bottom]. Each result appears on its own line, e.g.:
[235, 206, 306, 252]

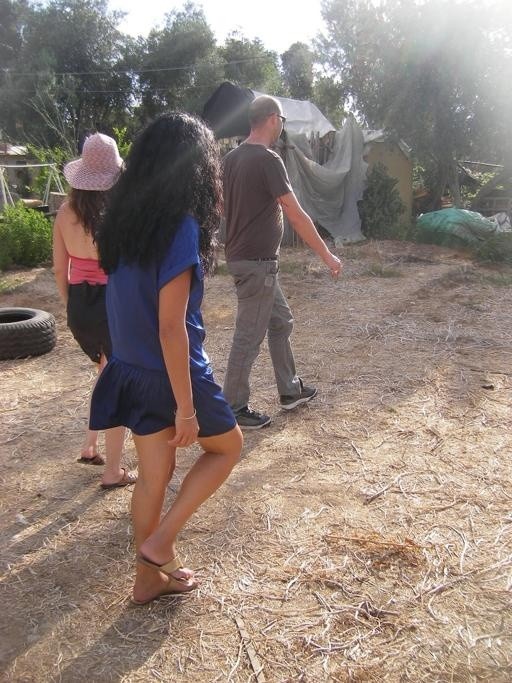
[268, 112, 287, 123]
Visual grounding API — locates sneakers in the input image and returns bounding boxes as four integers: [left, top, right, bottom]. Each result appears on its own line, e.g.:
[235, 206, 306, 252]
[279, 377, 318, 411]
[234, 405, 272, 430]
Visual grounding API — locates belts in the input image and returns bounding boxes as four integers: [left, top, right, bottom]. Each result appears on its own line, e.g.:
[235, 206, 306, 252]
[247, 255, 279, 262]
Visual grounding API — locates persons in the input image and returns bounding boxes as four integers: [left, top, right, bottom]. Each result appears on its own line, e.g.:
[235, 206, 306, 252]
[90, 108, 245, 607]
[52, 132, 138, 490]
[223, 92, 345, 429]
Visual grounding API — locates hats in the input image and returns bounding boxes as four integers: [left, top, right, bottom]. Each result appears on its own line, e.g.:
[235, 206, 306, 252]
[62, 131, 128, 193]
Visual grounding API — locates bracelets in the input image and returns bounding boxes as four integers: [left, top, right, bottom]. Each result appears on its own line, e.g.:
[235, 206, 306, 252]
[174, 408, 197, 420]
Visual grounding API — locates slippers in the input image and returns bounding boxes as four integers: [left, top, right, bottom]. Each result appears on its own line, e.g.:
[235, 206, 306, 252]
[76, 453, 107, 467]
[136, 555, 195, 587]
[130, 575, 199, 606]
[100, 467, 138, 490]
